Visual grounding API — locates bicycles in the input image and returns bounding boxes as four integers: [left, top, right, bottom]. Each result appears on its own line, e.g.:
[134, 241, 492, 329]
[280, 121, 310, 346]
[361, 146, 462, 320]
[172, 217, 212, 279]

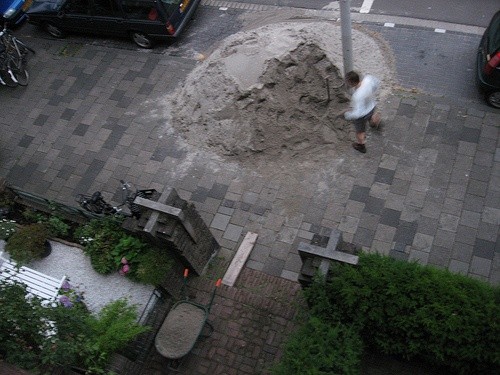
[0, 26, 36, 89]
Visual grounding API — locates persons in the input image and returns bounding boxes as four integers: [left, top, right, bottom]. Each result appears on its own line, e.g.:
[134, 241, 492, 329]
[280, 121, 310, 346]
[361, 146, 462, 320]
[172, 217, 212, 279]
[342, 71, 381, 153]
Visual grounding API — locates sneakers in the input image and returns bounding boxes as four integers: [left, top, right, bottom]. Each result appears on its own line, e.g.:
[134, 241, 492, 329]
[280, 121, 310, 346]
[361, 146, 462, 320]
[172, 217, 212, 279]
[352, 142, 367, 153]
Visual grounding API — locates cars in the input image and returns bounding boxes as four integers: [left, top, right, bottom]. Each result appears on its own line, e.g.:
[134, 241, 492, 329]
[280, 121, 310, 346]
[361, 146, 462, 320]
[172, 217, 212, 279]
[476, 9, 500, 109]
[26, 0, 201, 49]
[0, 1, 35, 33]
